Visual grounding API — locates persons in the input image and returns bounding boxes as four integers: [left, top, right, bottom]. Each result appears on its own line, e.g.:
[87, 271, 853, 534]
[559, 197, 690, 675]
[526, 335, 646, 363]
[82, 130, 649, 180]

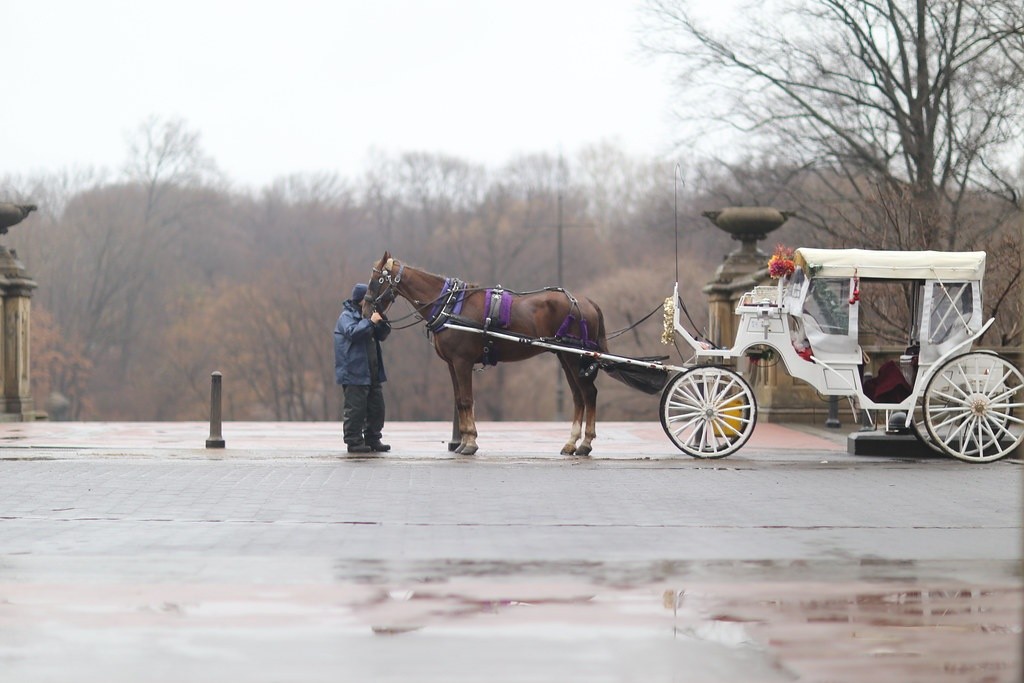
[334, 283, 392, 452]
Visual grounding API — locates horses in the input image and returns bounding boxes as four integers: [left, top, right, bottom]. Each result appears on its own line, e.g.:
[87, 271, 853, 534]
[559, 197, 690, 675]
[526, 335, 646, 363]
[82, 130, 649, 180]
[362, 250, 610, 456]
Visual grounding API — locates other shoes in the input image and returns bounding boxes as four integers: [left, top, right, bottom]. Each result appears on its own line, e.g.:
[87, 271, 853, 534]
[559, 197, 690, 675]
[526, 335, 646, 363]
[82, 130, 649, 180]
[366, 440, 391, 451]
[348, 444, 368, 452]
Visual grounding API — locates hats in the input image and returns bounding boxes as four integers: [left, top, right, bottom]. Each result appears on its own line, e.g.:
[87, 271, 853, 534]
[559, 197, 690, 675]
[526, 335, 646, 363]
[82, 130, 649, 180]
[351, 283, 368, 304]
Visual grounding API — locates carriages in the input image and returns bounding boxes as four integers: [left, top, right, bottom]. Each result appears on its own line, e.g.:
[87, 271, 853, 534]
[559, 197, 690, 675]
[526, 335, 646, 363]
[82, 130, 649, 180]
[361, 245, 1024, 464]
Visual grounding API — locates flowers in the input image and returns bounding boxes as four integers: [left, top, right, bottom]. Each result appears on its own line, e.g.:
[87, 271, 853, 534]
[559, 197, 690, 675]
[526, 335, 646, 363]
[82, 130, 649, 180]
[767, 245, 795, 279]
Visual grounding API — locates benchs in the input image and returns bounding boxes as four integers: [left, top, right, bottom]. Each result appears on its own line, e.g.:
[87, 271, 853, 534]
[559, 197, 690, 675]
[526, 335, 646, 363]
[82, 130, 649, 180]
[898, 312, 972, 394]
[730, 265, 802, 351]
